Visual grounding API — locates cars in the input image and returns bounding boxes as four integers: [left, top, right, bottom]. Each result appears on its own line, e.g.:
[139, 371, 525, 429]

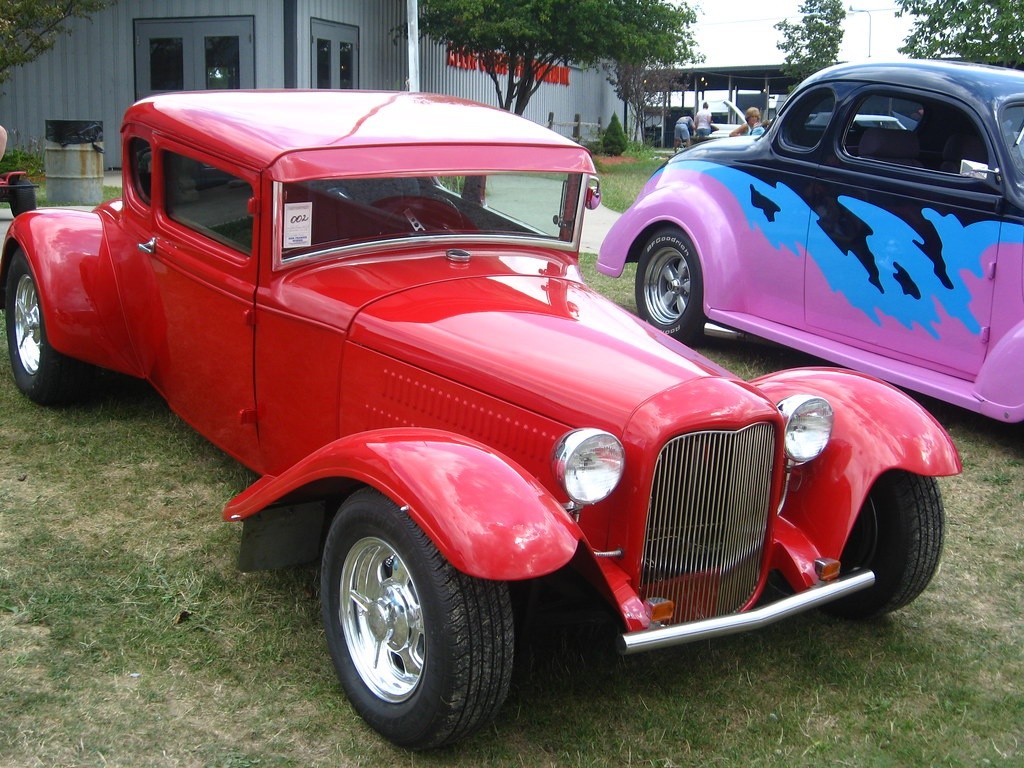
[0, 88, 964, 756]
[594, 58, 1024, 425]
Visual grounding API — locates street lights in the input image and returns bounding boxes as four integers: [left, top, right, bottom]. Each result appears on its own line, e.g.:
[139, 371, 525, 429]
[848, 5, 872, 59]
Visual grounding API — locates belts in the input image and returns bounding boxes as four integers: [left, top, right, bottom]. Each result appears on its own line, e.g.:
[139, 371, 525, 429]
[676, 122, 687, 124]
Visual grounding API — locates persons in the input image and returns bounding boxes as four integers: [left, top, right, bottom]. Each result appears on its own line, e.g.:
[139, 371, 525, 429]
[694, 102, 712, 143]
[728, 106, 764, 137]
[674, 116, 696, 154]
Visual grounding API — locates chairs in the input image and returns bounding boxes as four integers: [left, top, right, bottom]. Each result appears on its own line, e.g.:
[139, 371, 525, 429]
[857, 126, 924, 166]
[941, 132, 989, 173]
[300, 178, 421, 202]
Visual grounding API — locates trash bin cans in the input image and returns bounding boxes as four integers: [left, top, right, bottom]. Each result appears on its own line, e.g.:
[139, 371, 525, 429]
[42, 116, 106, 206]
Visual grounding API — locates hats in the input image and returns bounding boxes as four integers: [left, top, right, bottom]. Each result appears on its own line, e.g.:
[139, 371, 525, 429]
[745, 107, 763, 119]
